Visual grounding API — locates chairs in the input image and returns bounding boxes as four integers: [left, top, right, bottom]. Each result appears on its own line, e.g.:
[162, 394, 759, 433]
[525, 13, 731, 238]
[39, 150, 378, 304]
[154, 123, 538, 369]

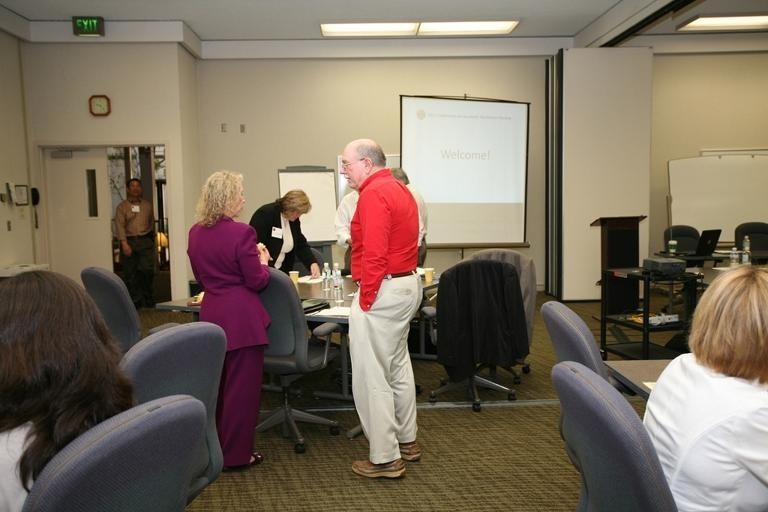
[734, 219, 767, 254]
[105, 318, 233, 507]
[21, 386, 210, 512]
[462, 246, 536, 384]
[426, 258, 533, 413]
[660, 225, 707, 312]
[540, 295, 637, 476]
[252, 266, 345, 456]
[545, 358, 684, 512]
[81, 267, 179, 351]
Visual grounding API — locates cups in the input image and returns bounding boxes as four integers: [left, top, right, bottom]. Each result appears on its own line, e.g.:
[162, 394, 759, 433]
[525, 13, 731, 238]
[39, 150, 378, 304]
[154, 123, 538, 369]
[423, 267, 434, 283]
[667, 240, 677, 253]
[294, 287, 300, 297]
[289, 270, 299, 287]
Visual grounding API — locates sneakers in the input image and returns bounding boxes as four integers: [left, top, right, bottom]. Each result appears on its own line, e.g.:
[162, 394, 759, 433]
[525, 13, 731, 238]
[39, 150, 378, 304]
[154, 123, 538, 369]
[351, 442, 422, 478]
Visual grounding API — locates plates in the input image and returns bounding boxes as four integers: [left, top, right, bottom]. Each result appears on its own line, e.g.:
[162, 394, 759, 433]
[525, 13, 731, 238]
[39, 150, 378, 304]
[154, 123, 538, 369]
[297, 274, 323, 285]
[414, 265, 426, 276]
[345, 274, 354, 278]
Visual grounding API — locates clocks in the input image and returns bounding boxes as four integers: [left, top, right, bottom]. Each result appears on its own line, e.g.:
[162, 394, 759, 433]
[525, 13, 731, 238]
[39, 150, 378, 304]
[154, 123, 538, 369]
[88, 94, 110, 116]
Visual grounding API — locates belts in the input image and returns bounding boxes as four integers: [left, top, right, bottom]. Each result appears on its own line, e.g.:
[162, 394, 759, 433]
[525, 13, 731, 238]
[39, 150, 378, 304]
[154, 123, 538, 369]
[384, 268, 417, 279]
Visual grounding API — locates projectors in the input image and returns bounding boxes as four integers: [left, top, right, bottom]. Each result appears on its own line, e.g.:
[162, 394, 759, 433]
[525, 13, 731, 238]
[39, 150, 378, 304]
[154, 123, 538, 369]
[642, 256, 687, 276]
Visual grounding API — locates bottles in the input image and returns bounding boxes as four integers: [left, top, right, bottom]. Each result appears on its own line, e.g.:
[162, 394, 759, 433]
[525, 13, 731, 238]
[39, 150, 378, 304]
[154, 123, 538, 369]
[332, 288, 344, 308]
[321, 289, 331, 308]
[729, 234, 752, 268]
[330, 262, 344, 289]
[320, 262, 331, 291]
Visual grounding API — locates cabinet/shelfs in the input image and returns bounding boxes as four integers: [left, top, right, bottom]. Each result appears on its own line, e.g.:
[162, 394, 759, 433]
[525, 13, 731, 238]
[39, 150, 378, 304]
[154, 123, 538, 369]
[599, 266, 703, 365]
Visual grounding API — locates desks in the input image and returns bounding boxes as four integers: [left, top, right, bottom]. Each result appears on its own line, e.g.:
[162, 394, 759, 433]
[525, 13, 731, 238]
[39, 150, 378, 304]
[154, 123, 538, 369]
[152, 270, 443, 408]
[663, 245, 767, 268]
[597, 357, 682, 402]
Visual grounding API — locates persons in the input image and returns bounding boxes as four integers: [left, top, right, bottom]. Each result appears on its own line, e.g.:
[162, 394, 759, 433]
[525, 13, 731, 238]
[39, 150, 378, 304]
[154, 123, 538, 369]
[391, 166, 427, 276]
[340, 135, 424, 477]
[1, 271, 136, 510]
[246, 188, 322, 281]
[115, 178, 159, 308]
[184, 170, 271, 471]
[643, 266, 765, 511]
[335, 187, 360, 276]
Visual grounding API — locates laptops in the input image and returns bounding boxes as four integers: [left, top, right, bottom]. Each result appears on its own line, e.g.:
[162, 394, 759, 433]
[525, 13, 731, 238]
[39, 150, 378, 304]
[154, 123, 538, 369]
[673, 229, 722, 257]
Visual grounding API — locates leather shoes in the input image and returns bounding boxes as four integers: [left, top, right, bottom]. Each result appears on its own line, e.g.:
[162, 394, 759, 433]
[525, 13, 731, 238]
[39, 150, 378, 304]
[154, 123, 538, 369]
[222, 451, 264, 472]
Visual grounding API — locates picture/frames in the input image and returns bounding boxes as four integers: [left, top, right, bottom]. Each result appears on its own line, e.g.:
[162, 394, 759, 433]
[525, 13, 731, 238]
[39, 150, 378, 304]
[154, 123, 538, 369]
[14, 184, 30, 207]
